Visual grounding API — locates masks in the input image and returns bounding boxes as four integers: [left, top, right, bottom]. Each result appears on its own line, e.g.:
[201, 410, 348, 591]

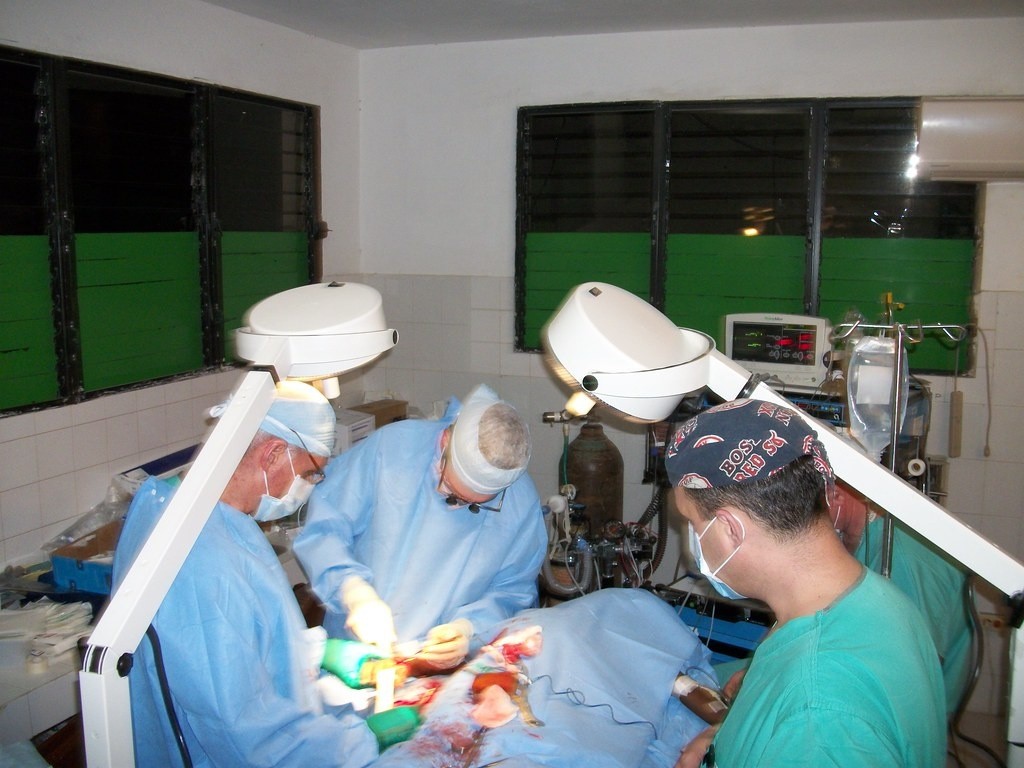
[689, 513, 749, 600]
[249, 448, 316, 522]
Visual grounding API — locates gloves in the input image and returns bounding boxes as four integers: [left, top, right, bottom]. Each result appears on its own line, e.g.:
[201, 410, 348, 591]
[321, 637, 387, 689]
[344, 585, 397, 645]
[414, 618, 473, 669]
[364, 707, 422, 755]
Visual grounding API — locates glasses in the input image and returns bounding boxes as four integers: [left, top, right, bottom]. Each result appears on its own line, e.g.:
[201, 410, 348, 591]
[290, 428, 326, 484]
[436, 458, 506, 514]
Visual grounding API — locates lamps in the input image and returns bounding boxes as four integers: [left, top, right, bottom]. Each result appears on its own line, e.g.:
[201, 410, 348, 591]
[540, 282, 1023, 768]
[76, 283, 400, 768]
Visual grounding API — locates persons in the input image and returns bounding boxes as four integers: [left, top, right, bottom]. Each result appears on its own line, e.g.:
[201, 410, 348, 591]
[666, 398, 974, 768]
[111, 378, 426, 768]
[292, 384, 548, 679]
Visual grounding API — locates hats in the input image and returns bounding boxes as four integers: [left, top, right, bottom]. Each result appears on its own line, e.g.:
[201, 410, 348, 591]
[665, 399, 833, 510]
[210, 378, 336, 457]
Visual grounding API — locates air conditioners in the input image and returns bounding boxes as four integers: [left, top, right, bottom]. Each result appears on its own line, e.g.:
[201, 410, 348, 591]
[914, 97, 1024, 182]
